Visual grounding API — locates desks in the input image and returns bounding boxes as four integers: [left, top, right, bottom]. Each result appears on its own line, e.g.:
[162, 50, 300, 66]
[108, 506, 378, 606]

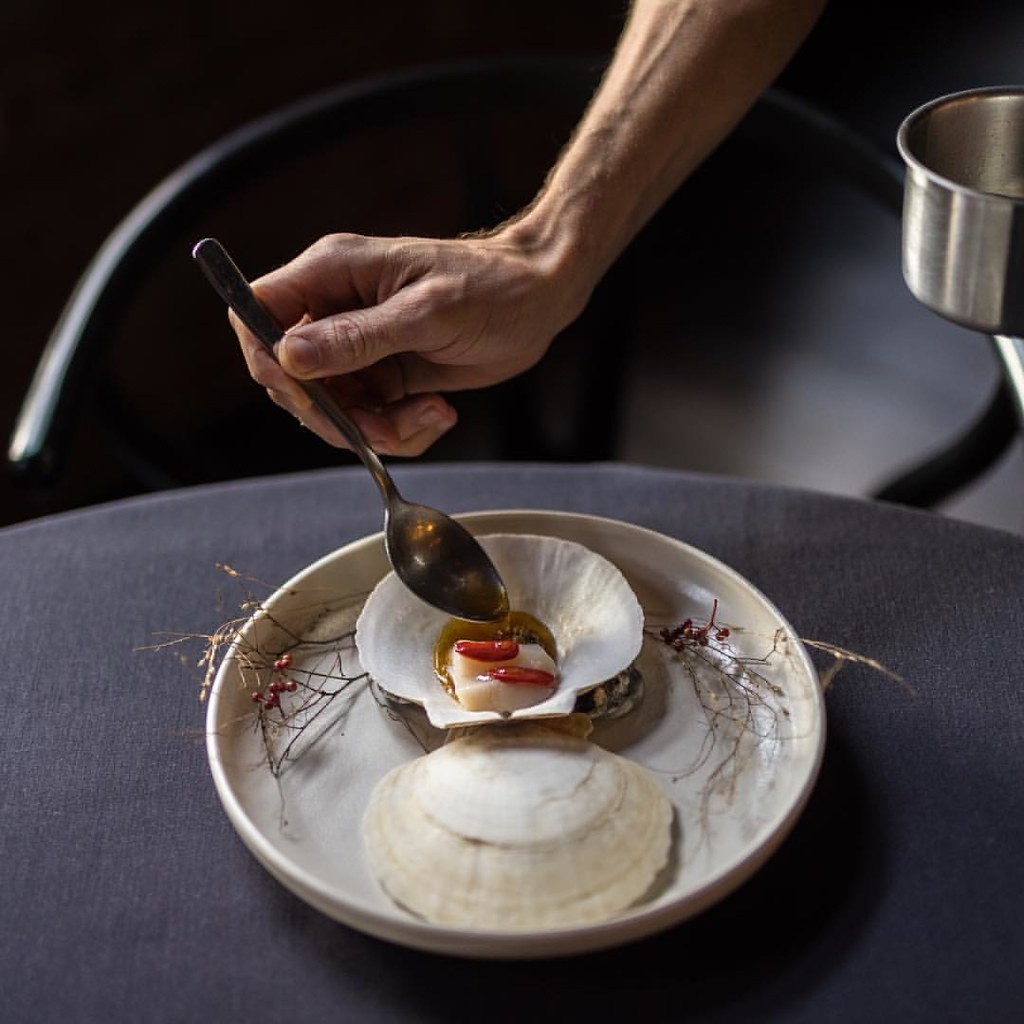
[0, 462, 1024, 1023]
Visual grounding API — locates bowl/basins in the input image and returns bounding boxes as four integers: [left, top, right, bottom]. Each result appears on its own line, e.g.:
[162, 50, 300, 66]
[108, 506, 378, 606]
[897, 85, 1024, 339]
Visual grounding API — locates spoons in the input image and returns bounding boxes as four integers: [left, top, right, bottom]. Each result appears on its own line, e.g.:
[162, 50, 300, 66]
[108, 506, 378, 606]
[192, 238, 511, 625]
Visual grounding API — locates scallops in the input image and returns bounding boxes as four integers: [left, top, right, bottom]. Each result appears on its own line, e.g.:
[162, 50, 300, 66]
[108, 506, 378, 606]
[353, 534, 675, 929]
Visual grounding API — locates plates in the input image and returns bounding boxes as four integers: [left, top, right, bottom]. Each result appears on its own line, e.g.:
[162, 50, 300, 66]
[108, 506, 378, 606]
[204, 506, 827, 959]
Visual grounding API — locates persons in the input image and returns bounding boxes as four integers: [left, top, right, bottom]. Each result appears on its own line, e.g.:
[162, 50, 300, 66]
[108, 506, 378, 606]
[226, 1, 818, 458]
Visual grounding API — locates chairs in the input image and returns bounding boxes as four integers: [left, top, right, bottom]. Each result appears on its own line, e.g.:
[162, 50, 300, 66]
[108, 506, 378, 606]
[8, 54, 1024, 509]
